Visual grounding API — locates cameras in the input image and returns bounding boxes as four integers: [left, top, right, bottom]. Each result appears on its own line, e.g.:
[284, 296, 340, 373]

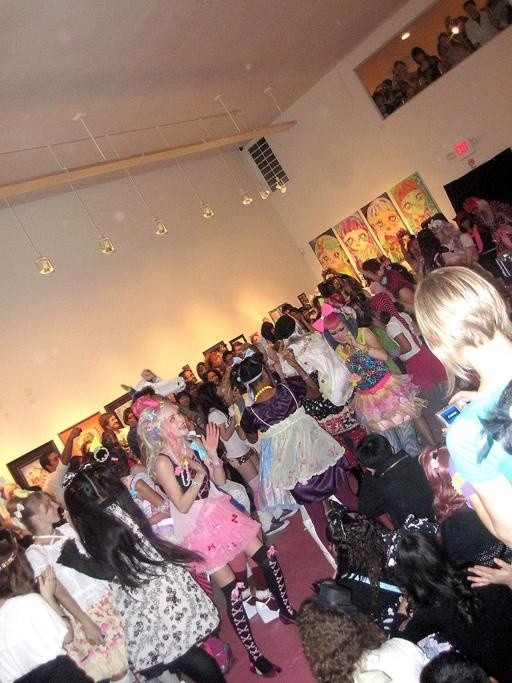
[431, 397, 468, 428]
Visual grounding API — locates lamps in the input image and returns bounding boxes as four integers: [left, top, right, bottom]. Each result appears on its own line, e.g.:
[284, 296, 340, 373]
[1, 97, 296, 275]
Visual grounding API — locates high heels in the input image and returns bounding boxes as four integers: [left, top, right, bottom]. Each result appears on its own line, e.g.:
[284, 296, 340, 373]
[242, 587, 256, 619]
[213, 643, 236, 674]
[280, 612, 297, 624]
[256, 589, 280, 624]
[250, 657, 281, 677]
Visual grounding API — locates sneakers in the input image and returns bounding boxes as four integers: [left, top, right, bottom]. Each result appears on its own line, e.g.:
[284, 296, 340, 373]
[277, 509, 298, 522]
[265, 520, 290, 536]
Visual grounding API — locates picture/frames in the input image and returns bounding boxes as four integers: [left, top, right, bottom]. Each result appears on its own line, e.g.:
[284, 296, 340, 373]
[59, 411, 105, 457]
[105, 392, 136, 429]
[6, 440, 62, 489]
[230, 335, 247, 349]
[269, 303, 291, 324]
[203, 340, 228, 358]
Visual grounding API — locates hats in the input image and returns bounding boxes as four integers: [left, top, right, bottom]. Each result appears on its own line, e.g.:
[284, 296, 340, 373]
[441, 510, 505, 565]
[272, 315, 295, 340]
[311, 583, 355, 608]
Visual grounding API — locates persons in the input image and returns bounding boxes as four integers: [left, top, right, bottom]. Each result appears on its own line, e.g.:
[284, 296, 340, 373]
[1, 196, 511, 682]
[371, 0, 511, 119]
